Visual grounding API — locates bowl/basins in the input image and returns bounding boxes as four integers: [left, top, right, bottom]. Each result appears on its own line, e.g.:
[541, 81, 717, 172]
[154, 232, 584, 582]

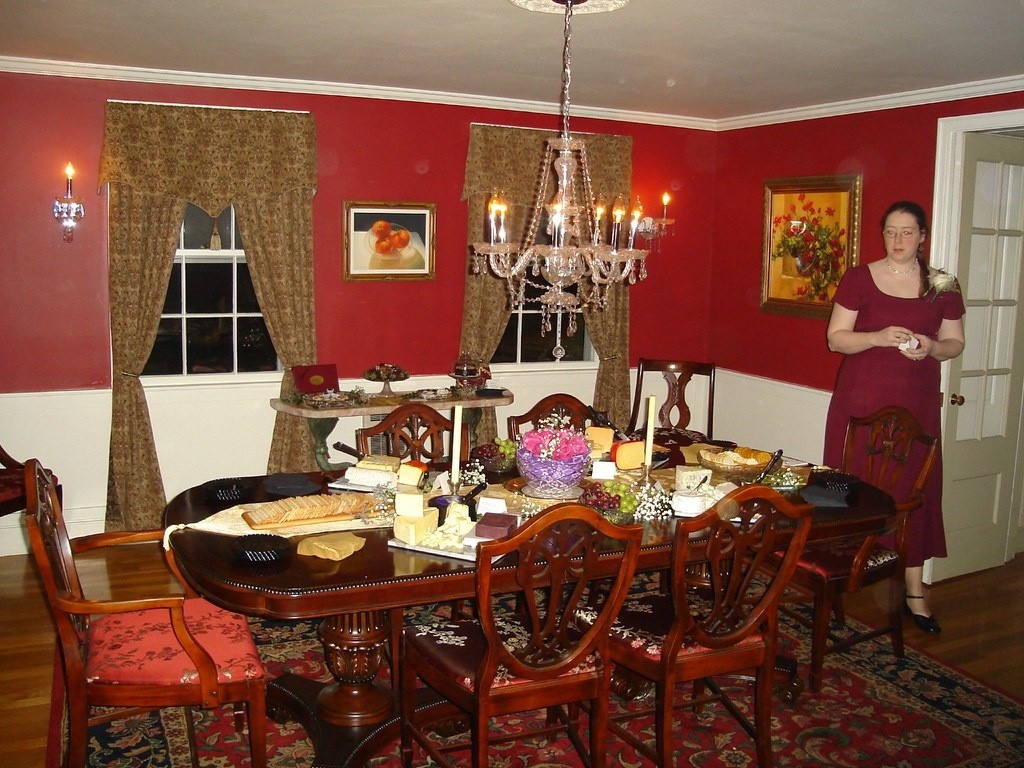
[671, 491, 706, 513]
[697, 447, 783, 486]
[470, 448, 518, 476]
[364, 223, 413, 260]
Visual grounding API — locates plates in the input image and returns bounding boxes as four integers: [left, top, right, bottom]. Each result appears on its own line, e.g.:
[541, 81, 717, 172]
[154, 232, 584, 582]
[387, 515, 523, 564]
[781, 456, 808, 467]
[302, 391, 360, 409]
[327, 472, 444, 493]
[242, 500, 395, 530]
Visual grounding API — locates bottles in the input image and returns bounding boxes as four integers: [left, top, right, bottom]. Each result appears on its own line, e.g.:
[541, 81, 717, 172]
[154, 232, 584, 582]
[456, 377, 479, 400]
[455, 352, 480, 376]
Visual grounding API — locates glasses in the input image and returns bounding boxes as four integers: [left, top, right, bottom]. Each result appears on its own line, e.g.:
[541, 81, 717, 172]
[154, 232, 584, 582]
[882, 229, 920, 238]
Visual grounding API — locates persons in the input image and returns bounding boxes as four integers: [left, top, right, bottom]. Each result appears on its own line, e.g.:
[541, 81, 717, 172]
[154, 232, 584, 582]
[823, 201, 966, 634]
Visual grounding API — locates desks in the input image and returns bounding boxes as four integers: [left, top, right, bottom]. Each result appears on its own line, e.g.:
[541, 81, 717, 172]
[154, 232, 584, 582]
[160, 441, 898, 768]
[270, 386, 514, 471]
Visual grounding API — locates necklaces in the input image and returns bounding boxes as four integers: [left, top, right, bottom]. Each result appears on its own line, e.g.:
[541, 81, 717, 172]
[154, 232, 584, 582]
[885, 257, 918, 275]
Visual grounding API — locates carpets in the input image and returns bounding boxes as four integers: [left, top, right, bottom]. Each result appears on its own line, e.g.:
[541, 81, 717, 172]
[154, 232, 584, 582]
[47, 558, 1024, 768]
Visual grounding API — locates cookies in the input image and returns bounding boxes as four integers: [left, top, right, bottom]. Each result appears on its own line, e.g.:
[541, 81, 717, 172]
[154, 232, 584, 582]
[247, 492, 380, 525]
[699, 447, 771, 465]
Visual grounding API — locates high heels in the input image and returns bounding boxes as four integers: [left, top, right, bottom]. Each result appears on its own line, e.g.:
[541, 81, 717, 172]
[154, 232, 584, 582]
[903, 594, 941, 634]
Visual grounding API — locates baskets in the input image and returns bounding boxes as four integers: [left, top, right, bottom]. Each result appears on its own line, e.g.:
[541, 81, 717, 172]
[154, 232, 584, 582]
[698, 448, 783, 485]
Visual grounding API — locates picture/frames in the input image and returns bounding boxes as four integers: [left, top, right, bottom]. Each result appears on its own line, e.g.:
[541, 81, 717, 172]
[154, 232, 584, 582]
[759, 173, 863, 319]
[341, 199, 437, 280]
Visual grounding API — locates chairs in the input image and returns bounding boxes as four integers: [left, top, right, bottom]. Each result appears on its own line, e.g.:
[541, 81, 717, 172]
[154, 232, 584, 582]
[25, 458, 267, 768]
[569, 485, 814, 767]
[508, 392, 609, 442]
[401, 502, 645, 768]
[624, 357, 716, 444]
[0, 448, 63, 530]
[356, 402, 471, 465]
[755, 404, 938, 694]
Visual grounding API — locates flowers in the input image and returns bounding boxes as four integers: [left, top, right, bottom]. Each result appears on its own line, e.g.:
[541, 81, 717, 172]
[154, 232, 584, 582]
[922, 266, 960, 304]
[515, 407, 596, 461]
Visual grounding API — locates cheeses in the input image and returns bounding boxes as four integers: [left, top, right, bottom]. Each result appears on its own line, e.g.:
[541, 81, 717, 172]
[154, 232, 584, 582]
[394, 491, 440, 546]
[585, 425, 647, 470]
[422, 515, 475, 549]
[356, 454, 428, 487]
[673, 465, 740, 514]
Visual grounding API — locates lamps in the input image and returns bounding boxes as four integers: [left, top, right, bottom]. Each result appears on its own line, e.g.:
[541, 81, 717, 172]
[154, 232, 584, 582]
[470, 0, 676, 339]
[53, 161, 84, 243]
[638, 191, 674, 241]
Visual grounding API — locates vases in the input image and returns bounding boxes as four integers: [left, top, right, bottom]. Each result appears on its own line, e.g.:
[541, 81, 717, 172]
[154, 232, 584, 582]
[516, 447, 590, 493]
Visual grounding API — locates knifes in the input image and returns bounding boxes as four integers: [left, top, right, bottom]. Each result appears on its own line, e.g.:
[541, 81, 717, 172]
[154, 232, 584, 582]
[597, 413, 630, 442]
[333, 442, 397, 465]
[460, 483, 487, 504]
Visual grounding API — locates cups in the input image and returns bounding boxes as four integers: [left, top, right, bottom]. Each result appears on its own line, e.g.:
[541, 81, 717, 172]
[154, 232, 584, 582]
[675, 465, 712, 491]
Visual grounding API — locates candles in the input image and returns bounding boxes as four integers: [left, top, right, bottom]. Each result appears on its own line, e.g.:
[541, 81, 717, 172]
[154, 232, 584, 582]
[645, 394, 656, 466]
[452, 403, 463, 482]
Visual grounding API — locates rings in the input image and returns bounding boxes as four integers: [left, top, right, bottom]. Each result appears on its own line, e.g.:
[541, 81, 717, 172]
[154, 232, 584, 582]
[914, 359, 916, 361]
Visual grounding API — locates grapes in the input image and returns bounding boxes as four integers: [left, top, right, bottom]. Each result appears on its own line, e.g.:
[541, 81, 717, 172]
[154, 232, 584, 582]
[582, 480, 639, 514]
[476, 436, 517, 466]
